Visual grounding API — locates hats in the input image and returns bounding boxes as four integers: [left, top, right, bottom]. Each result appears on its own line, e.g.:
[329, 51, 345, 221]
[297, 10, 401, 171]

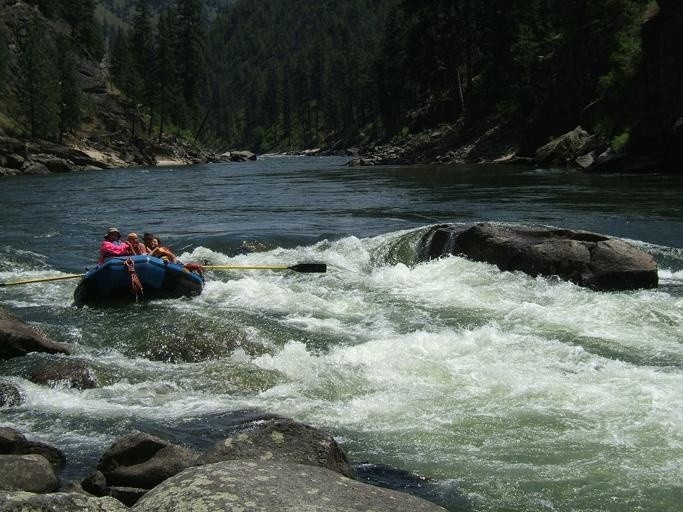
[142, 232, 154, 238]
[104, 228, 121, 238]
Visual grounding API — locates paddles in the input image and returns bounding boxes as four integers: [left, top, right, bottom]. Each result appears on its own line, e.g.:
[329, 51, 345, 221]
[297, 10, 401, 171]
[184, 264, 326, 272]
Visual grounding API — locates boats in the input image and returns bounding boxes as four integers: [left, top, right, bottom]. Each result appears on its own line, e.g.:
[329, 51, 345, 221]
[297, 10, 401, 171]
[74, 255, 205, 305]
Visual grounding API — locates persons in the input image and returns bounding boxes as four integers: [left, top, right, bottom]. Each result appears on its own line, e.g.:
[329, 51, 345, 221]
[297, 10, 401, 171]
[98, 227, 177, 264]
